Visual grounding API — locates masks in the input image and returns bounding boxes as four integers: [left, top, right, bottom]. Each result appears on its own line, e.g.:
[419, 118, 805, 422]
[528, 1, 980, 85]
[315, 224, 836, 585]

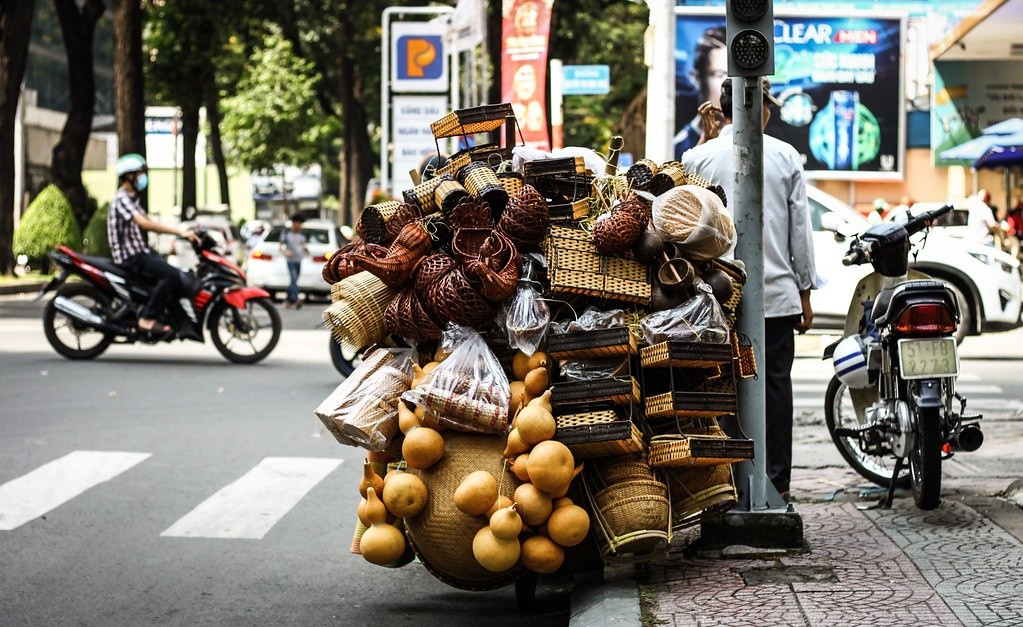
[130, 172, 147, 190]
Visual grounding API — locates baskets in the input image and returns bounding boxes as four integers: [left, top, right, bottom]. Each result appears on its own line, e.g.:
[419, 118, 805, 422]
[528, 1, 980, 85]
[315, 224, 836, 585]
[322, 144, 758, 590]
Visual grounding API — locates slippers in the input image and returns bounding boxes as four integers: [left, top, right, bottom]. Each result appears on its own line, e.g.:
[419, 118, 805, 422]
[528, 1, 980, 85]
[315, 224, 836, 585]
[140, 322, 172, 333]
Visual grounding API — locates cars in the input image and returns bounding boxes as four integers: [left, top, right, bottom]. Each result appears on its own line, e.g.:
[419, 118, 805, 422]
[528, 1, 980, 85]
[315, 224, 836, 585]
[804, 182, 1022, 347]
[172, 217, 354, 298]
[883, 198, 997, 243]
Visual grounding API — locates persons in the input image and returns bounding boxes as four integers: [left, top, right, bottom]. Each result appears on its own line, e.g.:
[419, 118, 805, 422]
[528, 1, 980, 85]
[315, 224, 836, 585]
[674, 24, 730, 162]
[279, 216, 310, 310]
[867, 187, 1023, 256]
[681, 75, 826, 500]
[106, 154, 202, 334]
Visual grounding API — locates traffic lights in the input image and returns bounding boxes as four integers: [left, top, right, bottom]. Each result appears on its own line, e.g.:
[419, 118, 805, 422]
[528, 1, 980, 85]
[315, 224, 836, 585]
[725, 0, 775, 78]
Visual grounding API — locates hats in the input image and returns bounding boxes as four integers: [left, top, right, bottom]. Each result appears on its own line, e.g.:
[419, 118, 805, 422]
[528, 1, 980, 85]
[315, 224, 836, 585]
[720, 74, 783, 108]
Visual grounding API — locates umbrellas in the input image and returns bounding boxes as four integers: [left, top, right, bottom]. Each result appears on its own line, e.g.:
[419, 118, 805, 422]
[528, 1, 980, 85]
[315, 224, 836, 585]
[945, 117, 1023, 214]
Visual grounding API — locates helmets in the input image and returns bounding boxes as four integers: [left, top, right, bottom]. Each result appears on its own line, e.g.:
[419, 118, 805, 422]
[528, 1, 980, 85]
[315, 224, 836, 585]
[116, 152, 146, 178]
[833, 332, 876, 389]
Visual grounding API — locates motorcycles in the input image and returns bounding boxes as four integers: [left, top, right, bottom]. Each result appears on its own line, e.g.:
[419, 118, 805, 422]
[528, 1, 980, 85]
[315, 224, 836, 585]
[33, 207, 283, 366]
[818, 202, 984, 513]
